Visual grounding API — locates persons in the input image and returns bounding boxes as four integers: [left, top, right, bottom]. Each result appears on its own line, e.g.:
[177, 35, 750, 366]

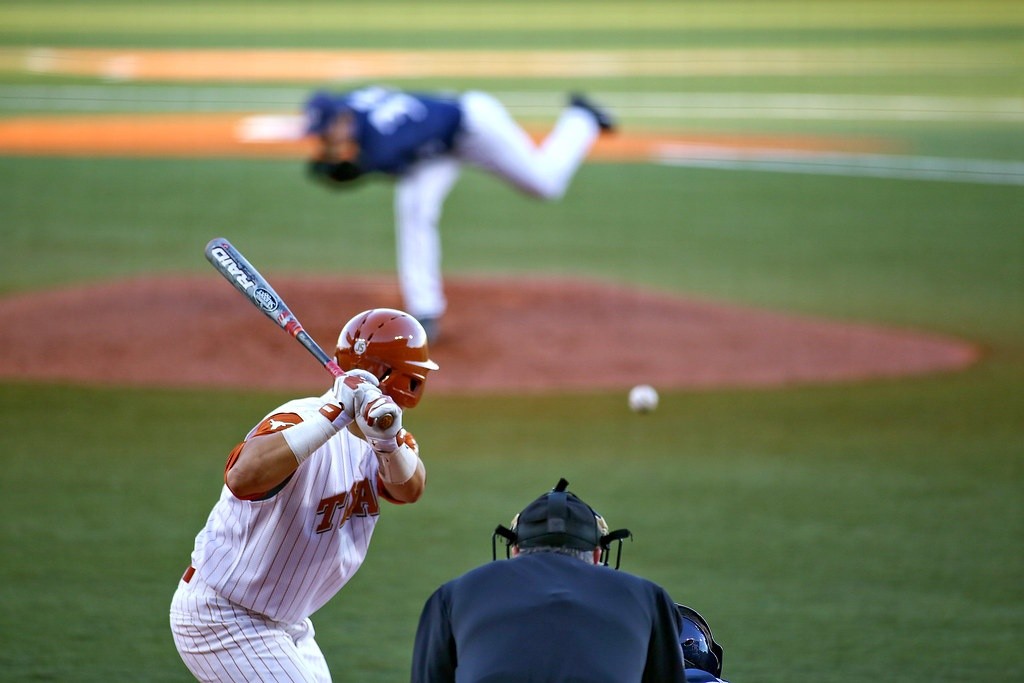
[302, 86, 622, 346]
[170, 308, 442, 683]
[411, 478, 729, 683]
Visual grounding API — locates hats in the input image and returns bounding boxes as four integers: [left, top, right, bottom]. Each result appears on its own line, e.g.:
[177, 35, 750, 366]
[492, 478, 634, 550]
[306, 92, 340, 136]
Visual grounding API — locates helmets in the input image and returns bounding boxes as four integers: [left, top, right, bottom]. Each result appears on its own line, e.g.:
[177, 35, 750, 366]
[334, 308, 439, 409]
[673, 603, 723, 676]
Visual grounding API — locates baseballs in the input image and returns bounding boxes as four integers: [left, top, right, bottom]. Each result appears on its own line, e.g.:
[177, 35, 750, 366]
[204, 235, 395, 430]
[627, 385, 659, 416]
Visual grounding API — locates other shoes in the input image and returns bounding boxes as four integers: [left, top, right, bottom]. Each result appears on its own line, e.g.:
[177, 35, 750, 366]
[571, 93, 611, 131]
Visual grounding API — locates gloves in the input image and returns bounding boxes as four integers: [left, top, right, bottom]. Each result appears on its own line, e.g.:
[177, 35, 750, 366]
[281, 369, 379, 466]
[354, 388, 417, 484]
